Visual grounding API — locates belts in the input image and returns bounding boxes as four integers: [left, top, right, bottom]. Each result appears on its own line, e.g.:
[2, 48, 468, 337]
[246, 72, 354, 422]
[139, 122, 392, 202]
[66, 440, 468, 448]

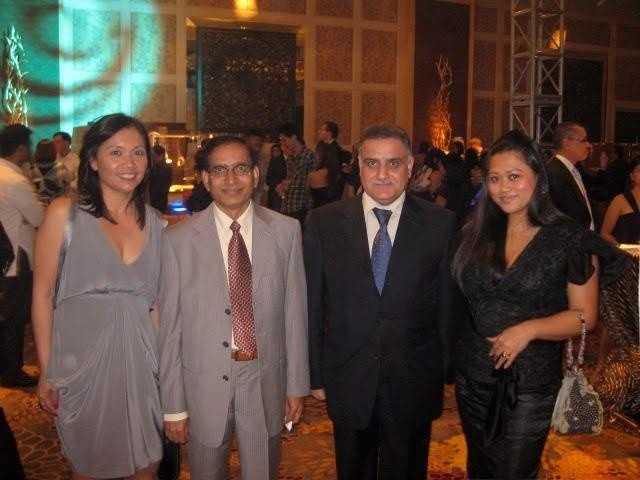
[230, 350, 257, 362]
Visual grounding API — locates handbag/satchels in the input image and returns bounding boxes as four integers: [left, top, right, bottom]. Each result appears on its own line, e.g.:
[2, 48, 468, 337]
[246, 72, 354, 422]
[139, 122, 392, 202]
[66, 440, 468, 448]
[553, 307, 605, 436]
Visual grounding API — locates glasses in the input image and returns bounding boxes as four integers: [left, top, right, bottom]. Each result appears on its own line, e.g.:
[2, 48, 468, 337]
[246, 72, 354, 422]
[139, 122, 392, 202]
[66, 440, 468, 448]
[569, 137, 587, 143]
[209, 163, 250, 177]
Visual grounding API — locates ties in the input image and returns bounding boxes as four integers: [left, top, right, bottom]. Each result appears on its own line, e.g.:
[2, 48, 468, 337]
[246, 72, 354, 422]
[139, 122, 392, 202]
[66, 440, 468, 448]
[226, 221, 260, 359]
[371, 208, 393, 295]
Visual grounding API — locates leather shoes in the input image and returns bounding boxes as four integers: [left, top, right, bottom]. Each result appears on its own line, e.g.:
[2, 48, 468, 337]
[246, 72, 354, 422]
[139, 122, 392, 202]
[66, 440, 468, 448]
[3, 371, 39, 388]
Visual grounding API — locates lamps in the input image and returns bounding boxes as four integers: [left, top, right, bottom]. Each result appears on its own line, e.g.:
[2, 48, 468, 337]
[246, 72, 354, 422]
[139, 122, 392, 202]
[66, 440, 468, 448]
[155, 134, 187, 167]
